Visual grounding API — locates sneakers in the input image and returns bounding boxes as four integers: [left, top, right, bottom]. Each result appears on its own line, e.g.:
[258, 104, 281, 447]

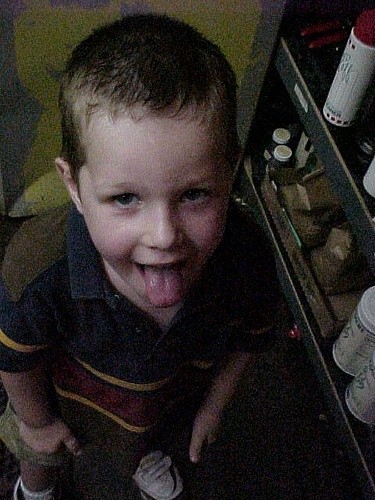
[134, 451, 183, 500]
[14, 475, 56, 500]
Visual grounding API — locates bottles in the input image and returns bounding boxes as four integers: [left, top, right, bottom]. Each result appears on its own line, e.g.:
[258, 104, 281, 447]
[263, 128, 291, 162]
[265, 145, 292, 175]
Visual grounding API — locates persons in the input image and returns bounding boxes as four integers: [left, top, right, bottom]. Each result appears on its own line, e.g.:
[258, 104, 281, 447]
[1, 12, 279, 499]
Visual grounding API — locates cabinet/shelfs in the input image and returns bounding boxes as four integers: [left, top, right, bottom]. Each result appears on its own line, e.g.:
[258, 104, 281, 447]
[231, 0, 375, 500]
